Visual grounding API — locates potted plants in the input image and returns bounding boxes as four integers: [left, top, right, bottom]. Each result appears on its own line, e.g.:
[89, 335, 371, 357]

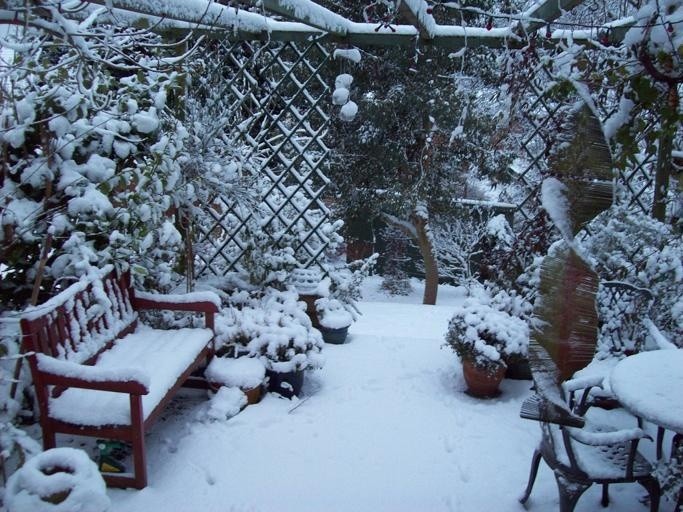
[447, 310, 503, 399]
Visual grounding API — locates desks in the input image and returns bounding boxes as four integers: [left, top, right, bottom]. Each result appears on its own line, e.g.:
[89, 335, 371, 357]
[612, 345, 683, 510]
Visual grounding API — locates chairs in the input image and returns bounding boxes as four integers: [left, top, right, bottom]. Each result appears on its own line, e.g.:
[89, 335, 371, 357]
[570, 274, 650, 429]
[517, 359, 661, 512]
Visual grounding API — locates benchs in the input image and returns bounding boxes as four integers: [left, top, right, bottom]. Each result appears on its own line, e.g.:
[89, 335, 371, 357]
[17, 265, 217, 488]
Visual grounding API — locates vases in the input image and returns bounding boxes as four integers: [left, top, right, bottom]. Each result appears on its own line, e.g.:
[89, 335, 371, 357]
[208, 358, 263, 403]
[268, 371, 302, 401]
[319, 321, 348, 344]
